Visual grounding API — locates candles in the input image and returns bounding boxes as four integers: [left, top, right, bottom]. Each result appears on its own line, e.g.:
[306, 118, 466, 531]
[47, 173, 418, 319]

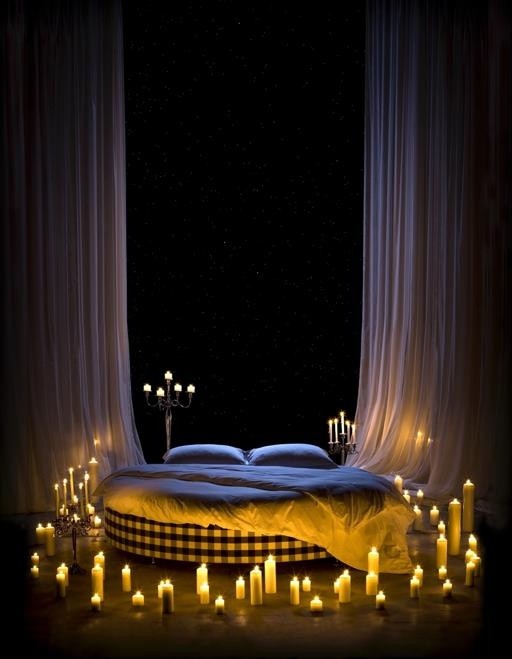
[328, 410, 356, 445]
[143, 371, 195, 397]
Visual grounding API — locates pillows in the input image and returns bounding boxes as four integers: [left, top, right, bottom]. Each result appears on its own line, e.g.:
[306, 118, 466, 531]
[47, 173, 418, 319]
[243, 443, 340, 469]
[163, 444, 247, 465]
